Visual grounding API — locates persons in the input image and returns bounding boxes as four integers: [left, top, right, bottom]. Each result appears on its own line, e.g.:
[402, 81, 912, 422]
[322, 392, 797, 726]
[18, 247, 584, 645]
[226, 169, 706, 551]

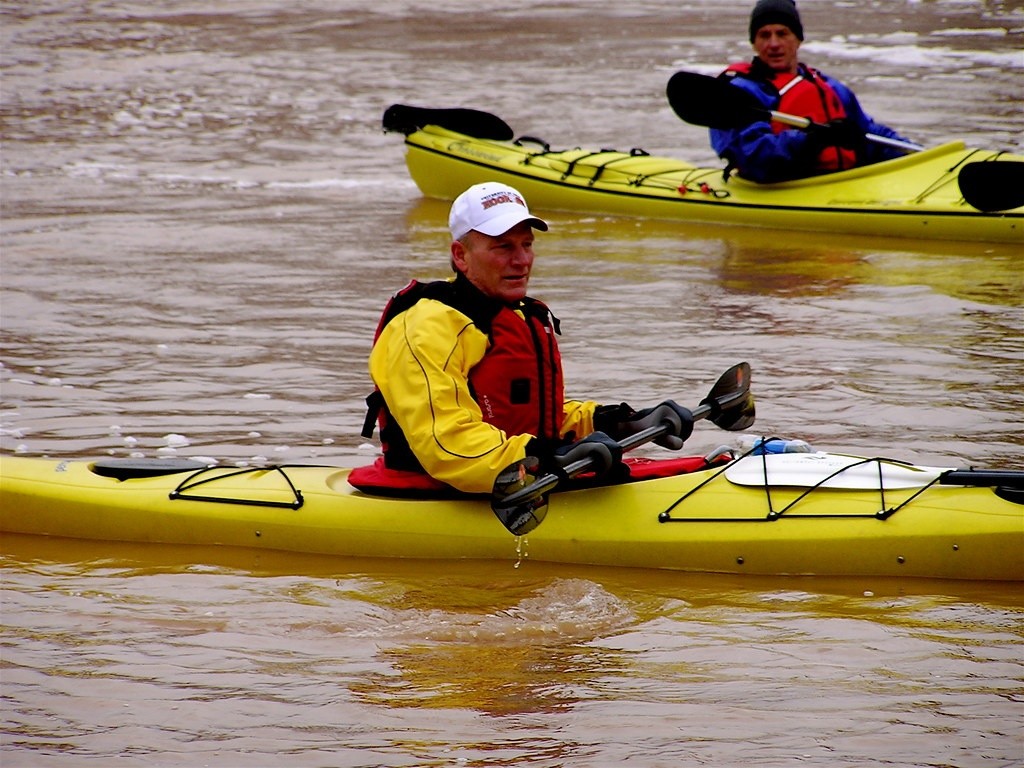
[360, 182, 694, 495]
[707, 0, 926, 183]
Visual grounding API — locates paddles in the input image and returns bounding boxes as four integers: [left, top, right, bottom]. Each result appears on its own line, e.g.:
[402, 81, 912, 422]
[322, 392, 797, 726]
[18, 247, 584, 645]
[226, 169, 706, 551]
[489, 362, 758, 538]
[665, 71, 925, 153]
[726, 451, 1024, 492]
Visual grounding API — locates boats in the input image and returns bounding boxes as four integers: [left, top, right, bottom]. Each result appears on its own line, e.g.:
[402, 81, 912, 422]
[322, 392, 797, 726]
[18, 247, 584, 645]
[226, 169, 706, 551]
[382, 104, 1024, 243]
[1, 436, 1024, 582]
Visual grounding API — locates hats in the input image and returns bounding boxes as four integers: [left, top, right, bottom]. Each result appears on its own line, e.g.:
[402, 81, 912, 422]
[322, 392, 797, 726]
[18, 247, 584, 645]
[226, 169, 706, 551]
[448, 182, 549, 244]
[749, 0, 804, 44]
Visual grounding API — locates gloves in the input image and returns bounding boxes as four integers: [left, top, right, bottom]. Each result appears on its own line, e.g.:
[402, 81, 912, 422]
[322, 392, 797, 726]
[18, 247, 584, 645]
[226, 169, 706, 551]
[546, 429, 621, 484]
[619, 398, 693, 451]
[812, 118, 866, 152]
